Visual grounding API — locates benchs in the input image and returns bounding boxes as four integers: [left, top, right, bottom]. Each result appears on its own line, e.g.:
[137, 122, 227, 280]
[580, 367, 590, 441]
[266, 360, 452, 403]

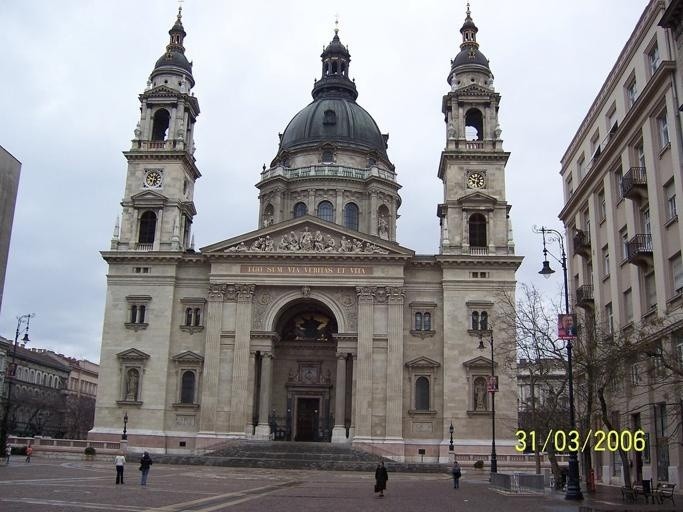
[621, 478, 644, 502]
[644, 482, 676, 507]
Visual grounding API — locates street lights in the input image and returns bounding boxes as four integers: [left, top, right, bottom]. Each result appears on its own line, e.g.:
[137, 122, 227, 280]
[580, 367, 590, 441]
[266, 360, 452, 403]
[121, 410, 129, 440]
[448, 419, 454, 451]
[2, 313, 33, 457]
[531, 221, 584, 504]
[478, 326, 500, 474]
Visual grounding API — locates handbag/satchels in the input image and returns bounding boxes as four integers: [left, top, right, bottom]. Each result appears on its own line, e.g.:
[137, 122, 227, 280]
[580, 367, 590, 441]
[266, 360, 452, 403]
[375, 485, 380, 492]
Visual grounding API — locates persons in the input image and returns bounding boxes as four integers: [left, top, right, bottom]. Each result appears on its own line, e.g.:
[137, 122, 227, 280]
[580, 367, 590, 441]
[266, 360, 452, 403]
[114, 451, 127, 484]
[451, 461, 460, 488]
[25, 445, 32, 463]
[127, 371, 137, 395]
[140, 451, 152, 485]
[562, 315, 577, 334]
[6, 444, 12, 464]
[375, 462, 388, 497]
[224, 227, 386, 253]
[475, 380, 486, 408]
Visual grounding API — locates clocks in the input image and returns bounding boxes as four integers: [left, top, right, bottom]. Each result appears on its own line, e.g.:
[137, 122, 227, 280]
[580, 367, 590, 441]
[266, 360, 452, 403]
[144, 168, 163, 188]
[466, 169, 486, 189]
